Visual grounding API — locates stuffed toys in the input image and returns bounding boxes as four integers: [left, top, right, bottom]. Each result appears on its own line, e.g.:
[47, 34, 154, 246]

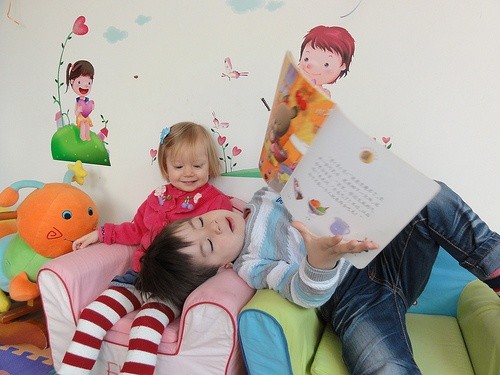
[0, 180, 99, 313]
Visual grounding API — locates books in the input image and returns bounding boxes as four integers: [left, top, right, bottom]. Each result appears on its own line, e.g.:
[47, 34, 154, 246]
[260, 51, 441, 269]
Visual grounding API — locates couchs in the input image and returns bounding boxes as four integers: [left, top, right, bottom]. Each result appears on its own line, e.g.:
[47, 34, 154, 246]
[37, 244, 500, 375]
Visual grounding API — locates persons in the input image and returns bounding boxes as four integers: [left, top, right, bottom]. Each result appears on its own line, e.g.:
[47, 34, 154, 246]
[54, 121, 233, 374]
[138, 181, 500, 375]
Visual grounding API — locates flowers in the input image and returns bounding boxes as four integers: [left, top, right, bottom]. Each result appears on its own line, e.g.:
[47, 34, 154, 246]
[160, 127, 170, 144]
